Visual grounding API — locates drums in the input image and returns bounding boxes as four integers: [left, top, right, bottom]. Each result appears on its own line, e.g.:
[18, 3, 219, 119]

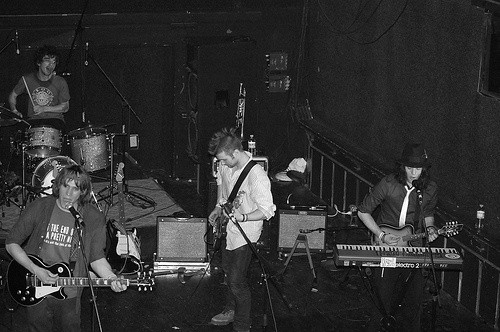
[68, 127, 112, 174]
[30, 156, 79, 197]
[22, 124, 64, 157]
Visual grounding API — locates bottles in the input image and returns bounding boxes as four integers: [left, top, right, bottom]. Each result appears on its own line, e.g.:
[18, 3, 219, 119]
[248, 135, 257, 156]
[474, 204, 486, 237]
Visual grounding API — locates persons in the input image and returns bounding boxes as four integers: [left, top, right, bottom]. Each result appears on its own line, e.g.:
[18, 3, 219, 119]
[206, 127, 274, 332]
[7, 45, 70, 124]
[356, 145, 440, 331]
[5, 164, 129, 332]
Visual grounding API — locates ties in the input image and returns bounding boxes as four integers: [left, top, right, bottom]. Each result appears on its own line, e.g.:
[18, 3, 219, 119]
[398, 185, 415, 228]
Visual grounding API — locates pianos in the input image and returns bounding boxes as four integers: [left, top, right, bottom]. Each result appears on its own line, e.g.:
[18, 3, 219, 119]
[333, 243, 463, 272]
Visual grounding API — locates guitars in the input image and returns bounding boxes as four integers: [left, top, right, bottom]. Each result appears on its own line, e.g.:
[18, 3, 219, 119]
[5, 254, 156, 306]
[112, 162, 144, 261]
[212, 190, 246, 251]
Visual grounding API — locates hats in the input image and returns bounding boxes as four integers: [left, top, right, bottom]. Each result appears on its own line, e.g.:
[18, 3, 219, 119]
[396, 143, 431, 169]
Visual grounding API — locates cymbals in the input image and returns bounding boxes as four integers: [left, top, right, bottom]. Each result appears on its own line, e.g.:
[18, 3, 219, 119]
[0, 106, 21, 128]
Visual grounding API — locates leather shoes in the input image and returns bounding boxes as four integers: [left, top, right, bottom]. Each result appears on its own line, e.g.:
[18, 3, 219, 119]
[211, 309, 236, 326]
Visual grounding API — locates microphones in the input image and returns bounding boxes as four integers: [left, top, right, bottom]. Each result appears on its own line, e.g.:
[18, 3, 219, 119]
[62, 200, 86, 228]
[412, 179, 425, 192]
[83, 41, 90, 66]
[14, 29, 22, 56]
[219, 197, 238, 224]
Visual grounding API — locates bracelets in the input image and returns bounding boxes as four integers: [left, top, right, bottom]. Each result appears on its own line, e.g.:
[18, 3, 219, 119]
[241, 214, 248, 222]
[378, 231, 387, 242]
